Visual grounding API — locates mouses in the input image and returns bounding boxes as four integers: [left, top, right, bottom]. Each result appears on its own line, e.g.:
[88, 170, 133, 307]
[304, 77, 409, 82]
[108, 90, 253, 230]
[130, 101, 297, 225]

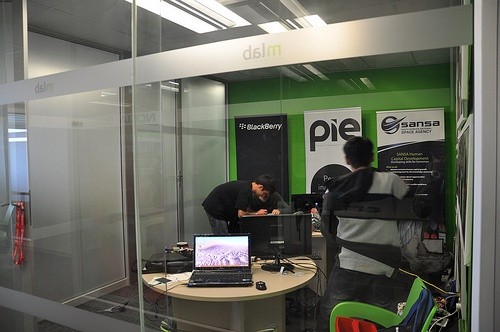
[256, 281, 266, 290]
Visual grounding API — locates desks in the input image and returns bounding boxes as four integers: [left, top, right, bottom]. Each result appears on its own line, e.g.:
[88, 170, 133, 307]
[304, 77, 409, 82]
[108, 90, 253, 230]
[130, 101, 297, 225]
[142, 256, 317, 332]
[308, 232, 326, 261]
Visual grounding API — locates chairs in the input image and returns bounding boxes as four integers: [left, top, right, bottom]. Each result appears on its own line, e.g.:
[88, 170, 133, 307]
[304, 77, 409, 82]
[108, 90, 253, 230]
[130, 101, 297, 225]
[330, 277, 438, 332]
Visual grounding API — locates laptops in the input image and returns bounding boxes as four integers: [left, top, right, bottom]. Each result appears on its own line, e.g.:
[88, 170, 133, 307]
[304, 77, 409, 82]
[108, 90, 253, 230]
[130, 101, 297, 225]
[145, 253, 191, 270]
[187, 233, 253, 287]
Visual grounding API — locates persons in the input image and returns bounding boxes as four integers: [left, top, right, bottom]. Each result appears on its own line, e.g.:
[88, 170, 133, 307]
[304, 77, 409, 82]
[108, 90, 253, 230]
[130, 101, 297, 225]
[320, 137, 413, 332]
[247, 185, 289, 215]
[201, 174, 274, 235]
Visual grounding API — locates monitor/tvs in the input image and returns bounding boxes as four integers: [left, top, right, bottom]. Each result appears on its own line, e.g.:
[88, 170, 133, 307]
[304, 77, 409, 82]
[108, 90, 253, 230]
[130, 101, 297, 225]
[241, 214, 312, 271]
[291, 193, 324, 216]
